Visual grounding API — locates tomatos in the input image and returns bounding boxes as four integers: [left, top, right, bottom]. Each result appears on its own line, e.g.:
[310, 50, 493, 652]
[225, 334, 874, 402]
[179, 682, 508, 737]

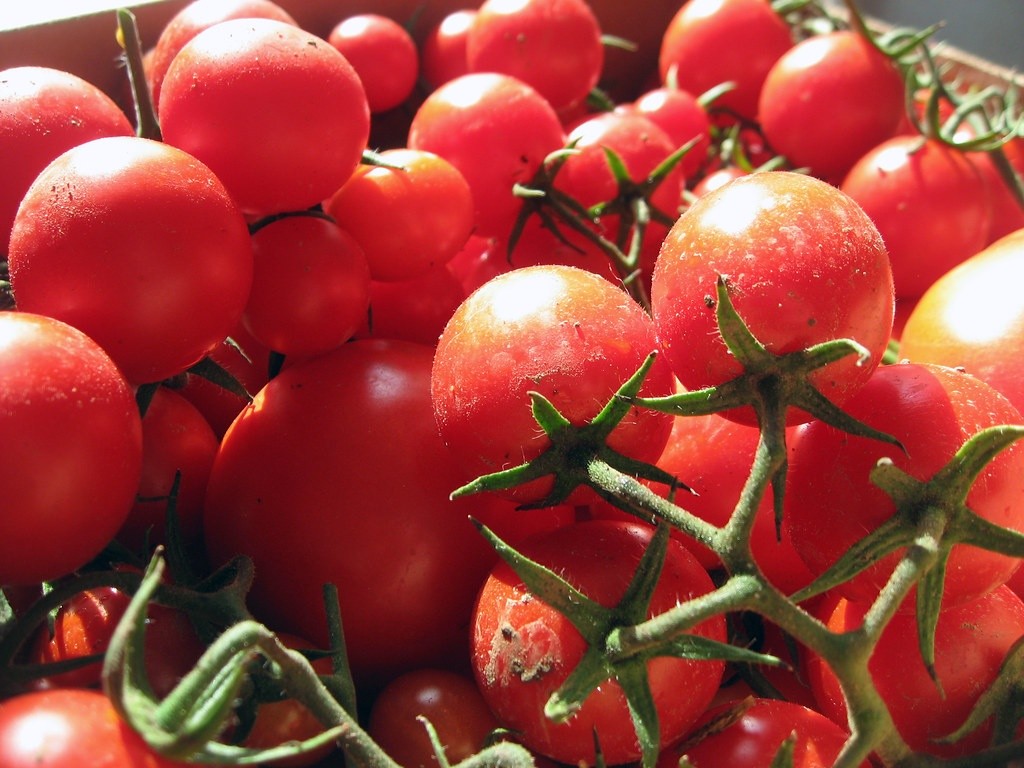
[0, 0, 1024, 768]
